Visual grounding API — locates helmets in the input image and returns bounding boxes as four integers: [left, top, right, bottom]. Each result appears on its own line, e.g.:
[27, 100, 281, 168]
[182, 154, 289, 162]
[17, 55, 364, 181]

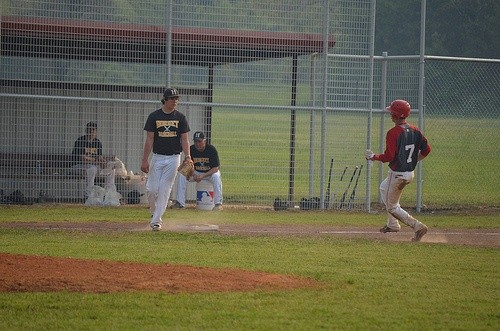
[385, 100, 410, 118]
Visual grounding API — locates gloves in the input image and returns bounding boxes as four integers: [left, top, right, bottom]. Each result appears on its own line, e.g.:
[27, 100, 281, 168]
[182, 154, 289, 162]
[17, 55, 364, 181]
[364, 150, 375, 161]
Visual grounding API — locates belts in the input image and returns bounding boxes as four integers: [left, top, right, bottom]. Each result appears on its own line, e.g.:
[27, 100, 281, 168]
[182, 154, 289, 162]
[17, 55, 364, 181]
[154, 152, 181, 156]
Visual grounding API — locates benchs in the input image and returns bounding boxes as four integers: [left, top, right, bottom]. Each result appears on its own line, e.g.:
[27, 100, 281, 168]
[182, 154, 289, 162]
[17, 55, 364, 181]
[0, 153, 130, 204]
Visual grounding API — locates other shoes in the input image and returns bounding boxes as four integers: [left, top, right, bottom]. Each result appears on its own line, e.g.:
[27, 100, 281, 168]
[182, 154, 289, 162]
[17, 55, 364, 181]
[380, 225, 400, 233]
[170, 202, 185, 208]
[152, 225, 161, 231]
[149, 214, 155, 223]
[411, 226, 428, 243]
[213, 204, 223, 211]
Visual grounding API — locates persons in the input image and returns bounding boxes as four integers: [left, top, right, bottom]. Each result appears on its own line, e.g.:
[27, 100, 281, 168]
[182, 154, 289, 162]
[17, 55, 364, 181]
[72, 120, 115, 197]
[170, 131, 223, 210]
[141, 88, 192, 231]
[364, 100, 431, 242]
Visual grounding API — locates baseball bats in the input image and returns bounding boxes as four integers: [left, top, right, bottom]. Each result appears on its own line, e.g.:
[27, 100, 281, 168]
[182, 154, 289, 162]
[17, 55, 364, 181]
[324, 158, 334, 210]
[331, 165, 349, 206]
[337, 165, 359, 210]
[348, 164, 363, 211]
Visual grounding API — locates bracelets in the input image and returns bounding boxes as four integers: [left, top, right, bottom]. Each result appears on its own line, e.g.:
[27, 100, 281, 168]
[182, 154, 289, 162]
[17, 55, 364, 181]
[185, 156, 191, 159]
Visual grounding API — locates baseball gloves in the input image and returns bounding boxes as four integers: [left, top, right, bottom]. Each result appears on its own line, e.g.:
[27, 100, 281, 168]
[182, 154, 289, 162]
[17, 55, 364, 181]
[176, 155, 194, 181]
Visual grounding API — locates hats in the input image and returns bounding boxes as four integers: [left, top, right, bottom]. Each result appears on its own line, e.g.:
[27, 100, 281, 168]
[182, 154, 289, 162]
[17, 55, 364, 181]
[192, 132, 206, 141]
[163, 88, 181, 98]
